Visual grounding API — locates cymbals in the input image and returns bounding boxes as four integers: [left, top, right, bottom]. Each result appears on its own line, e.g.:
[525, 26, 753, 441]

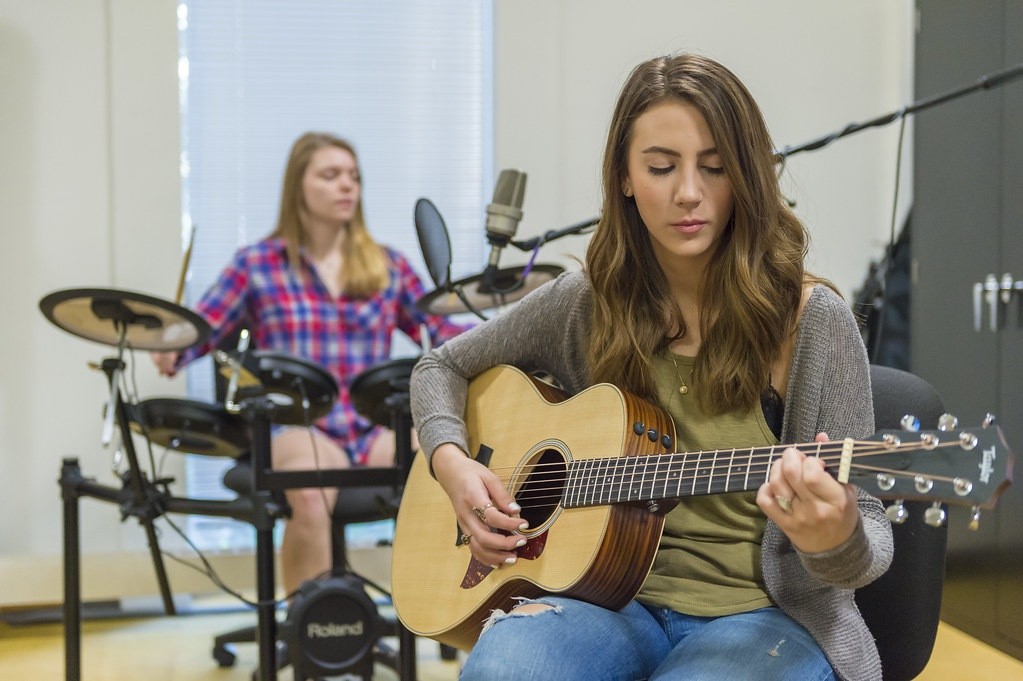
[37, 287, 215, 353]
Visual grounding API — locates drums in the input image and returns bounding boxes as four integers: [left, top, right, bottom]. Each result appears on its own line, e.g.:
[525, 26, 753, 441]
[349, 357, 421, 430]
[221, 352, 341, 426]
[130, 397, 251, 458]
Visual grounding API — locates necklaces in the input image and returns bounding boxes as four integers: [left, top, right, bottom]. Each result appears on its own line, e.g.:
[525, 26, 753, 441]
[668, 343, 695, 394]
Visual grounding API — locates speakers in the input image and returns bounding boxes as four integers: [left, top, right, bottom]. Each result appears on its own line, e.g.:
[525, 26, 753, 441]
[286, 573, 379, 681]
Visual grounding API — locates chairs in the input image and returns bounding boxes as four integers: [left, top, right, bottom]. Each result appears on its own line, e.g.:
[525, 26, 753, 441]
[212, 326, 457, 666]
[857, 367, 954, 681]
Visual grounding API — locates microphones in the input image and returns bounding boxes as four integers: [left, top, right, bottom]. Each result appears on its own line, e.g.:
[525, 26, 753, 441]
[480, 169, 528, 293]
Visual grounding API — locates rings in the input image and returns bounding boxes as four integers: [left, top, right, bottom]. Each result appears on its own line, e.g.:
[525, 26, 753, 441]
[461, 534, 473, 545]
[472, 503, 494, 524]
[776, 495, 797, 513]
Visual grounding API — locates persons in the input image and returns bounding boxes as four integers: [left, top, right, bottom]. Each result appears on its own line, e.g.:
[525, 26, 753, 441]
[151, 132, 486, 610]
[409, 54, 894, 681]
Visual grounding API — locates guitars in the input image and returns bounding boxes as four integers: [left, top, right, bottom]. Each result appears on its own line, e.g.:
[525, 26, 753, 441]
[388, 360, 1016, 653]
